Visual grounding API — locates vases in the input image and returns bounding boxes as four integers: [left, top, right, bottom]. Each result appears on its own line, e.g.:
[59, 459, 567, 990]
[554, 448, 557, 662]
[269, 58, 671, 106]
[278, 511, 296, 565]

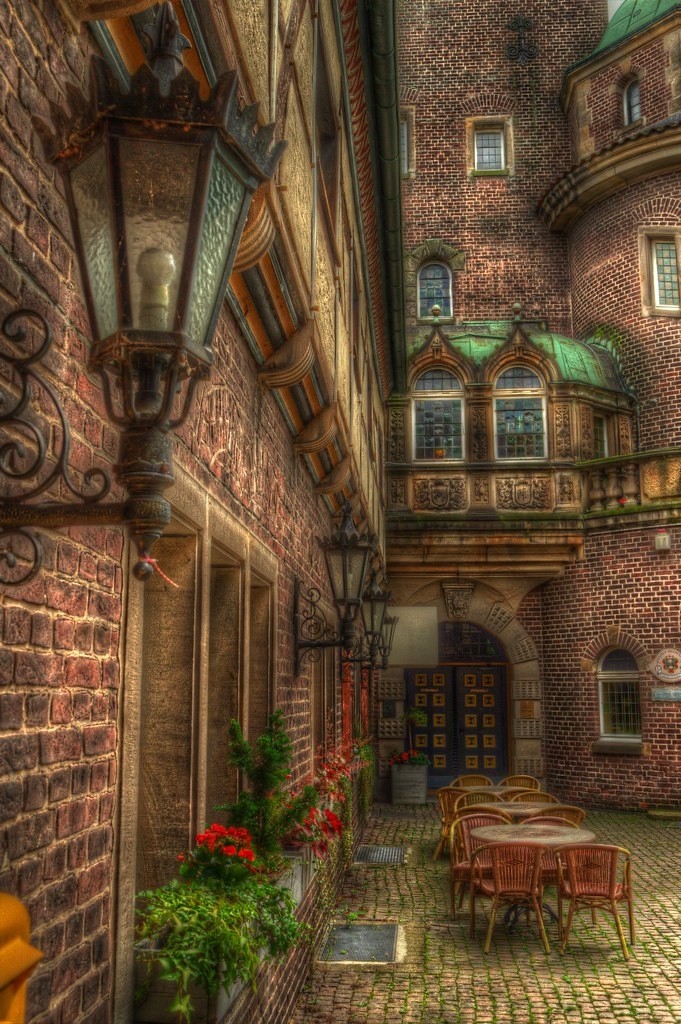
[390, 763, 428, 807]
[132, 786, 344, 1024]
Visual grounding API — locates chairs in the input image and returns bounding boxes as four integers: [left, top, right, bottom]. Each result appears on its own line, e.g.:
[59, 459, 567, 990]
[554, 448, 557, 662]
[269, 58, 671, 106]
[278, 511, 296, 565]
[432, 773, 637, 962]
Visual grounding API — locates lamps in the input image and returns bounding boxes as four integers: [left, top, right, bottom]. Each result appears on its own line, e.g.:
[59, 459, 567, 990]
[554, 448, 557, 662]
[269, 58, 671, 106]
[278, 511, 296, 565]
[0, 0, 289, 591]
[293, 498, 400, 681]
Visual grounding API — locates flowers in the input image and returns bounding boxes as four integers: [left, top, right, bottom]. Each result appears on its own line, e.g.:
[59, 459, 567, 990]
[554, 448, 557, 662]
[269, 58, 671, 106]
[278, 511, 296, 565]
[385, 747, 431, 767]
[265, 733, 374, 863]
[175, 823, 256, 885]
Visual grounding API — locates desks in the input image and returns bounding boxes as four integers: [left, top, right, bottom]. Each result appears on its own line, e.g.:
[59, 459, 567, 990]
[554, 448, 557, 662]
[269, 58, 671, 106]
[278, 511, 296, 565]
[457, 785, 529, 795]
[471, 801, 567, 819]
[469, 824, 590, 937]
